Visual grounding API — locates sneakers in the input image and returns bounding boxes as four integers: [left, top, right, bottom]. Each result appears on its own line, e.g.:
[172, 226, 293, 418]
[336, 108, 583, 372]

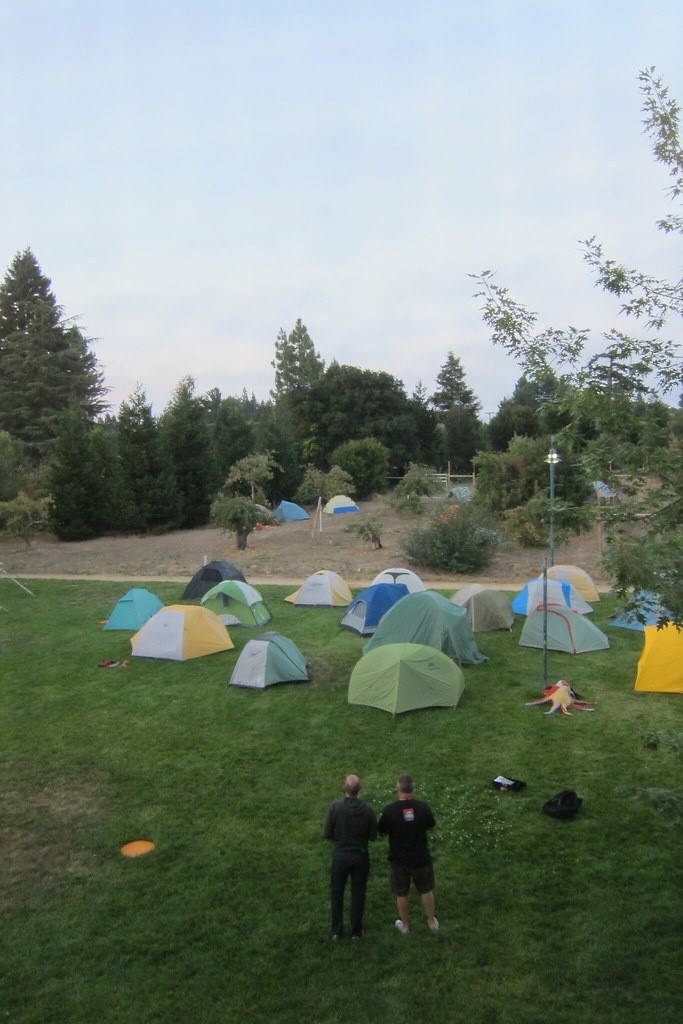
[428, 917, 439, 932]
[395, 919, 409, 935]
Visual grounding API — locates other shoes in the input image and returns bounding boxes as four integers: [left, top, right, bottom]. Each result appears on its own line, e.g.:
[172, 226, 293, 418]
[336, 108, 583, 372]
[333, 934, 344, 943]
[352, 929, 365, 944]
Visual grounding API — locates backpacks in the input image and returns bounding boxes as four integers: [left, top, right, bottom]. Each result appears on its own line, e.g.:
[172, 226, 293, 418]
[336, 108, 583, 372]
[544, 789, 581, 820]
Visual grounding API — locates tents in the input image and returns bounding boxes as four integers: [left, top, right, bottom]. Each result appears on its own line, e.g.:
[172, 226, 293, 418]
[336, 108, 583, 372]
[361, 592, 490, 667]
[634, 620, 682, 694]
[201, 580, 275, 632]
[371, 567, 427, 594]
[272, 500, 310, 522]
[104, 588, 163, 632]
[518, 602, 612, 655]
[347, 643, 468, 717]
[539, 564, 601, 602]
[227, 631, 313, 691]
[452, 584, 515, 632]
[511, 576, 594, 615]
[341, 582, 409, 639]
[606, 587, 676, 632]
[129, 603, 236, 662]
[282, 569, 354, 609]
[446, 486, 472, 504]
[181, 560, 249, 600]
[323, 495, 361, 516]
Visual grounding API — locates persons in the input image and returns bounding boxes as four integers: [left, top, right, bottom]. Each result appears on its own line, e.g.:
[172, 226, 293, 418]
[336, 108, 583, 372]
[377, 775, 440, 933]
[322, 775, 378, 943]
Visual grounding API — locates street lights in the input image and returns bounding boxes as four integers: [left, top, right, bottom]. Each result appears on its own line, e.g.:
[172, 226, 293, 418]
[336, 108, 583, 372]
[542, 435, 563, 689]
